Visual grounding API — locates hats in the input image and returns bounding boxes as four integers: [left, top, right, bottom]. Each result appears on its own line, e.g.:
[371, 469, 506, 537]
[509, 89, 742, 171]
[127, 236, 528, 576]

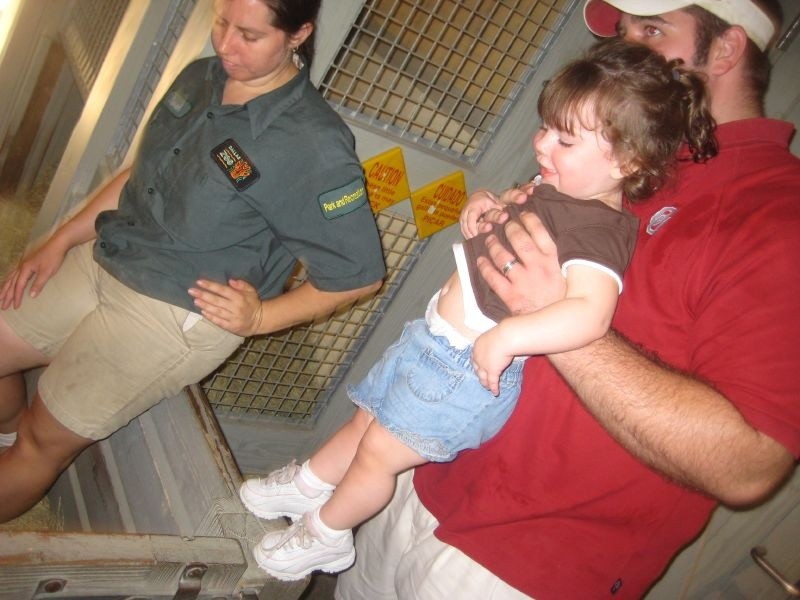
[583, 0, 783, 55]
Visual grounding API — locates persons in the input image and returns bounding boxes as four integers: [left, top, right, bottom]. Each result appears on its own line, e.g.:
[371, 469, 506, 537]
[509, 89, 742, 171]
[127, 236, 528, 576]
[240, 38, 718, 582]
[0, 0, 384, 523]
[335, 0, 800, 600]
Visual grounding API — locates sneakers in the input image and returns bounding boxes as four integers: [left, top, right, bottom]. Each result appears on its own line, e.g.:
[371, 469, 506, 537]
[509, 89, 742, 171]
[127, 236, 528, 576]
[252, 512, 358, 581]
[238, 463, 334, 521]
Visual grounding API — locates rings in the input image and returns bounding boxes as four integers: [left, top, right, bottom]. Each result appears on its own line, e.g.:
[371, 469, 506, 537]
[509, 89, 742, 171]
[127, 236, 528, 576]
[502, 257, 520, 277]
